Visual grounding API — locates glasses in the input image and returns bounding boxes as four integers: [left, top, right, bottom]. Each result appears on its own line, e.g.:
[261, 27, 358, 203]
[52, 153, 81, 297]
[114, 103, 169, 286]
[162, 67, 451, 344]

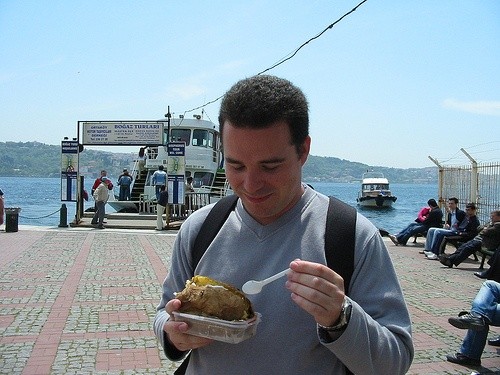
[466, 209, 472, 211]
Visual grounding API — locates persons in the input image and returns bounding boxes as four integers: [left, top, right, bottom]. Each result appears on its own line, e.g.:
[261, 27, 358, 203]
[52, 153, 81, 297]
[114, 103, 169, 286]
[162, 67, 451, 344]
[0, 188, 4, 225]
[388, 198, 444, 246]
[91, 177, 110, 229]
[419, 197, 467, 253]
[151, 165, 167, 200]
[91, 170, 114, 212]
[154, 75, 415, 375]
[178, 177, 195, 217]
[445, 280, 500, 366]
[116, 168, 133, 201]
[473, 244, 500, 283]
[137, 147, 147, 179]
[439, 210, 500, 267]
[424, 203, 480, 259]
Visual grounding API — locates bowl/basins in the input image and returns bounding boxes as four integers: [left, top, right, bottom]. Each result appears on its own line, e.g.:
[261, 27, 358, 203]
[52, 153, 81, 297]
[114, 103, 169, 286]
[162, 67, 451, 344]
[171, 310, 263, 345]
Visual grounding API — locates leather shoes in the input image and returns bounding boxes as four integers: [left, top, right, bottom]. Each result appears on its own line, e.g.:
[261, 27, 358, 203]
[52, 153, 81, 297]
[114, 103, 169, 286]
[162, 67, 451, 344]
[474, 267, 497, 280]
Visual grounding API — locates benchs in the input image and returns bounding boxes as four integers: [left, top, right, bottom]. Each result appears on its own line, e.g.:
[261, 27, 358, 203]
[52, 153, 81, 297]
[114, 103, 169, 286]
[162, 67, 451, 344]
[413, 219, 480, 262]
[477, 248, 494, 270]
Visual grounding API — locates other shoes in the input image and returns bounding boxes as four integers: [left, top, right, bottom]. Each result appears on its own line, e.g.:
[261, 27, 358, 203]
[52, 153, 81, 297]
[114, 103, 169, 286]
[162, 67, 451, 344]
[155, 228, 162, 230]
[98, 225, 105, 228]
[91, 220, 98, 224]
[103, 221, 107, 223]
[388, 234, 399, 246]
[488, 335, 500, 344]
[439, 255, 453, 268]
[424, 251, 433, 255]
[419, 251, 424, 254]
[427, 253, 438, 260]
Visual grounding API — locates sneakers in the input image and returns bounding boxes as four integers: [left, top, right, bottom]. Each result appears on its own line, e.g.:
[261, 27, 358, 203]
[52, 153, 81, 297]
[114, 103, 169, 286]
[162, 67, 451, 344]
[446, 351, 481, 367]
[448, 311, 485, 331]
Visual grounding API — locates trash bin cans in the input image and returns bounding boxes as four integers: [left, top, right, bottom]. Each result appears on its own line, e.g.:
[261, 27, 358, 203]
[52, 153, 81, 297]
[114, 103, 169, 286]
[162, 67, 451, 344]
[4, 207, 20, 232]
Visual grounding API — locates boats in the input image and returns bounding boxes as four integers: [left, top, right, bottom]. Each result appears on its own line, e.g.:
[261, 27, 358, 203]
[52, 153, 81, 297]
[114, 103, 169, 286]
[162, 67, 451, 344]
[105, 104, 235, 215]
[356, 169, 397, 210]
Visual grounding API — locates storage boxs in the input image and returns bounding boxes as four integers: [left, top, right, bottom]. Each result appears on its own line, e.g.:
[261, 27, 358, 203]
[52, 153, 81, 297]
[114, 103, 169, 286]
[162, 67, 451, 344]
[172, 309, 262, 345]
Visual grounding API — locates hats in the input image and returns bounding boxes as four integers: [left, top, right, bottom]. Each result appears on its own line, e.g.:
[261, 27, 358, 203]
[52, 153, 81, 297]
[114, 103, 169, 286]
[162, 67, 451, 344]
[102, 177, 109, 181]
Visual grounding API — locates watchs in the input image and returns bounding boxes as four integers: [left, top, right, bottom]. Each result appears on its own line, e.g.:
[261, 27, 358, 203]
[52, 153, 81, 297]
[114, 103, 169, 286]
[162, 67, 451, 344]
[318, 296, 352, 333]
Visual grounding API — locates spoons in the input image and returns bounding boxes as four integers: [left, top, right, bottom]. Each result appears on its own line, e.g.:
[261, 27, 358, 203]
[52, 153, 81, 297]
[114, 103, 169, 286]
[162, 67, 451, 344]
[242, 268, 291, 295]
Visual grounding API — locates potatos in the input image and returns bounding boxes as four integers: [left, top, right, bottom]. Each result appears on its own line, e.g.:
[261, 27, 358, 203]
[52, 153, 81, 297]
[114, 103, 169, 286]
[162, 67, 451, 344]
[174, 274, 254, 338]
